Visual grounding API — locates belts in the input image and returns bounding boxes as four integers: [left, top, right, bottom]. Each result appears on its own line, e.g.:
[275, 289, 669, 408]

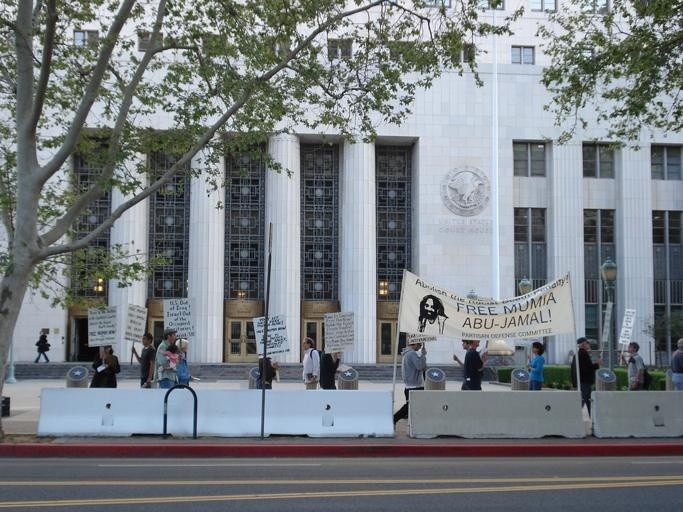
[673, 371, 683, 373]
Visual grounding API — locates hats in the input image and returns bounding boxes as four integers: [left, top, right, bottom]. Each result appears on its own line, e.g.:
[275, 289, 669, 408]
[577, 338, 586, 344]
[163, 329, 178, 337]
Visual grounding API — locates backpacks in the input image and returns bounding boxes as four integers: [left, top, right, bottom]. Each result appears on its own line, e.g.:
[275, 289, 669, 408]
[310, 350, 322, 359]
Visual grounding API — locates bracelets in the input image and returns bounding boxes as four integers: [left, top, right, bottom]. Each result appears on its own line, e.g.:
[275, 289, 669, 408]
[636, 378, 640, 382]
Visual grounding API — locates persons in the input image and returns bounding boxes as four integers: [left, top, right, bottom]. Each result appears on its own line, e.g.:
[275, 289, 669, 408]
[302, 337, 320, 390]
[670, 339, 683, 391]
[392, 342, 427, 432]
[571, 337, 604, 416]
[319, 351, 342, 390]
[619, 342, 645, 391]
[32, 330, 51, 363]
[460, 340, 488, 391]
[89, 333, 200, 389]
[416, 295, 449, 335]
[525, 341, 545, 391]
[452, 340, 483, 386]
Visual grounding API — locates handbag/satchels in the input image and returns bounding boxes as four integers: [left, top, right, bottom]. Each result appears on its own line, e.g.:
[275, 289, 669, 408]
[644, 369, 652, 389]
